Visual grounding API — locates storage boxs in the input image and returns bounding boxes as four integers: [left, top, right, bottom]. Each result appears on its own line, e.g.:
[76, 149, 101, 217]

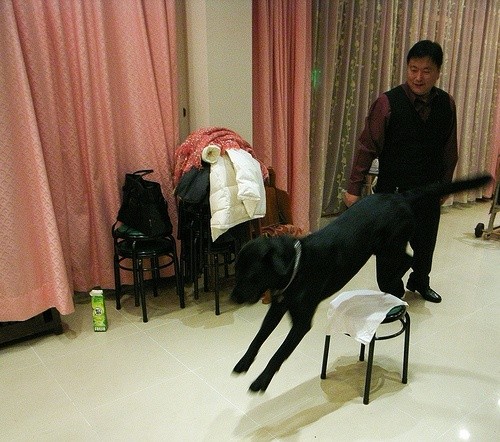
[89, 289, 108, 332]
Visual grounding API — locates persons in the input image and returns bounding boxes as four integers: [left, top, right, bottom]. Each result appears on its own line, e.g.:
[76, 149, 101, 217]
[343, 40, 458, 303]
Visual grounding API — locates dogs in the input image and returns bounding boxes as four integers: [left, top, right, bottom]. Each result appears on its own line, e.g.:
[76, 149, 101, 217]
[227, 174, 492, 393]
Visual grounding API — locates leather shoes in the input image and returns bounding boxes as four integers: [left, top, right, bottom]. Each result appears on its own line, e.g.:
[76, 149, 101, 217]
[406, 280, 442, 303]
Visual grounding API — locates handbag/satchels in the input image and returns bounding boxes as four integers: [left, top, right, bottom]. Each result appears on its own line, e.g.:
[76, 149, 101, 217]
[112, 170, 174, 247]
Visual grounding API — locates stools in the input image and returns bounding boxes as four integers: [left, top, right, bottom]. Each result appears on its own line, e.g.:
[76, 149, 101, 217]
[114, 192, 241, 322]
[320, 290, 410, 405]
